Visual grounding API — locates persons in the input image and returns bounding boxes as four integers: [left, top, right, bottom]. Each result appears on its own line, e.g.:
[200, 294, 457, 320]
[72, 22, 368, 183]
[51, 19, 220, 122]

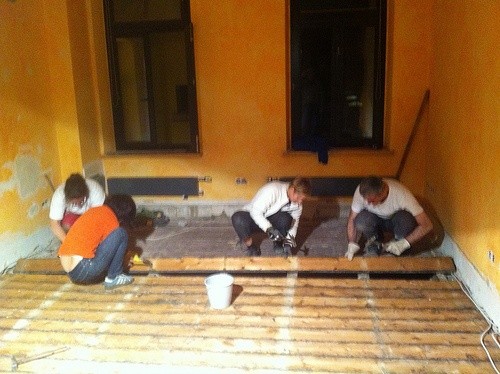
[345, 175, 433, 262]
[49, 173, 106, 244]
[231, 176, 313, 259]
[58, 193, 136, 290]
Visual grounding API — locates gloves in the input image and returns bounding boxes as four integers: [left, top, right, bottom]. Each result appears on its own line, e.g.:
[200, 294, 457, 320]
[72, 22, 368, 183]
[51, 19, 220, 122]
[385, 239, 411, 256]
[281, 244, 293, 258]
[344, 243, 360, 261]
[268, 228, 285, 241]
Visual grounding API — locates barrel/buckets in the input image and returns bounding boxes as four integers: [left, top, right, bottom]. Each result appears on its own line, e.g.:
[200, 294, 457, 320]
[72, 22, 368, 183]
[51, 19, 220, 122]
[204, 273, 234, 309]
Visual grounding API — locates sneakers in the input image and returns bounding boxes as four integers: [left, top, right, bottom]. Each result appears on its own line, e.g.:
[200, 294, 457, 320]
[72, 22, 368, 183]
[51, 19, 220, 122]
[272, 241, 284, 254]
[104, 273, 134, 290]
[244, 248, 261, 257]
